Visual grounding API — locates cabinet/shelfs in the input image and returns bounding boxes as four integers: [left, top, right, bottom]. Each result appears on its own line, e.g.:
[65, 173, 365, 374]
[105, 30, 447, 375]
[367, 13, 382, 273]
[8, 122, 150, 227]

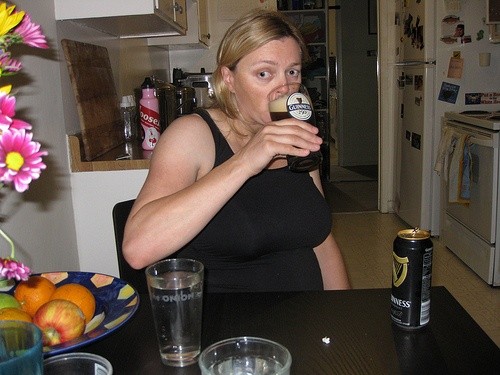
[275, 1, 331, 180]
[53, 0, 210, 50]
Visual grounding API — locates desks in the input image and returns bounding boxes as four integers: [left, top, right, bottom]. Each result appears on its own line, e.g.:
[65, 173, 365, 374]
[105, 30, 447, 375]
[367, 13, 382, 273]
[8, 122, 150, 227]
[44, 285, 500, 375]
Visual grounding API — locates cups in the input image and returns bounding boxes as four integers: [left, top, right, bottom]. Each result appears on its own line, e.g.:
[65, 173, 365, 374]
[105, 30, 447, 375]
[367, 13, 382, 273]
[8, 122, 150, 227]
[0, 321, 42, 375]
[177, 87, 194, 114]
[41, 352, 112, 375]
[266, 82, 320, 173]
[145, 258, 204, 367]
[198, 337, 291, 375]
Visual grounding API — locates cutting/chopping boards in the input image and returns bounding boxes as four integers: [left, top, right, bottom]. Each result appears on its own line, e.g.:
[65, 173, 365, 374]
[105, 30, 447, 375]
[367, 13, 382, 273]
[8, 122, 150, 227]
[61, 38, 126, 161]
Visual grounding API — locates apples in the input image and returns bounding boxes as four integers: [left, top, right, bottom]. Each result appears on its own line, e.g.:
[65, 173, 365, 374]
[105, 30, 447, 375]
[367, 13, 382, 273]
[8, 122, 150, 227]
[0, 292, 22, 309]
[32, 299, 86, 347]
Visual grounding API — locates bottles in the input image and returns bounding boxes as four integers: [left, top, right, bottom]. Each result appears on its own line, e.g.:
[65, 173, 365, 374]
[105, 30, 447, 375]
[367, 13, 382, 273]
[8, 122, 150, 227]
[191, 81, 208, 108]
[140, 77, 161, 150]
[120, 94, 138, 141]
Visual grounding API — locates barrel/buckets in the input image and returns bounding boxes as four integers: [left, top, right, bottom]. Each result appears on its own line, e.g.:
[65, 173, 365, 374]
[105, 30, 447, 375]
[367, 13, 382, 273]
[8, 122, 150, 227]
[134, 79, 177, 135]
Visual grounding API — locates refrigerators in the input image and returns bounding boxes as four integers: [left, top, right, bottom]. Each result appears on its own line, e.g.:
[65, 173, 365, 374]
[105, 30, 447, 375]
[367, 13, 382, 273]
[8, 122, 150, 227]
[395, 0, 500, 235]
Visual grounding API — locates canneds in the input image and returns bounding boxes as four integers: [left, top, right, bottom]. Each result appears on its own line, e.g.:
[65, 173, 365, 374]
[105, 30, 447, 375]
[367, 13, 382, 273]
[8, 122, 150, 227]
[391, 227, 434, 330]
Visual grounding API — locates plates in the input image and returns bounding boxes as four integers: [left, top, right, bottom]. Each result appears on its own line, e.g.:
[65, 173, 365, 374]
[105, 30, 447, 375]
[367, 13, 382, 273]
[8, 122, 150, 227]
[0, 271, 139, 355]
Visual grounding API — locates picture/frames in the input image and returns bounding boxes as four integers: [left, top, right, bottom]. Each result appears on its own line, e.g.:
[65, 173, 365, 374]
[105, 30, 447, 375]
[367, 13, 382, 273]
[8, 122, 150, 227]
[367, 0, 377, 35]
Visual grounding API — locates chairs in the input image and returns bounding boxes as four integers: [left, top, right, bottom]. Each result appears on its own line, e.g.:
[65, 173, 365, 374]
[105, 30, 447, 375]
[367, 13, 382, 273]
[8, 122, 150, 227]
[112, 200, 147, 287]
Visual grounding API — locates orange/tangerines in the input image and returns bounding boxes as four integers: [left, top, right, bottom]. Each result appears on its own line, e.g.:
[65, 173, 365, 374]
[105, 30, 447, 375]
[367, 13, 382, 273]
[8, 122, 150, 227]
[0, 275, 95, 348]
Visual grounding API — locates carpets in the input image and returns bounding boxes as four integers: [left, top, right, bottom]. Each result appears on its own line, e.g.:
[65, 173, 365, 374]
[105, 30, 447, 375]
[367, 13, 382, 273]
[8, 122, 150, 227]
[323, 165, 379, 208]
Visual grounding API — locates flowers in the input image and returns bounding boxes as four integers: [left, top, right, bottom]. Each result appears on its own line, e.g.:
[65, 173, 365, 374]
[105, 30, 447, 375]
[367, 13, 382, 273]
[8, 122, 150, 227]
[0, 0, 49, 281]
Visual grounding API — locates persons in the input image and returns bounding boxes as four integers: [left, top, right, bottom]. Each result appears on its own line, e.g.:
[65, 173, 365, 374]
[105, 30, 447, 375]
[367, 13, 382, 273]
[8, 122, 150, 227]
[121, 10, 349, 296]
